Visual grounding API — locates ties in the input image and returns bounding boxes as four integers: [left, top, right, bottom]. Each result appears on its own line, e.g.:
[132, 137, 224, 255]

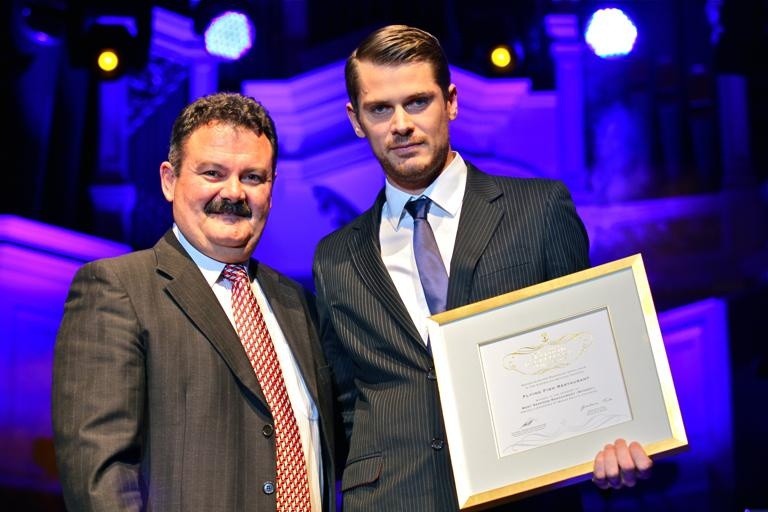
[404, 196, 450, 348]
[222, 261, 312, 512]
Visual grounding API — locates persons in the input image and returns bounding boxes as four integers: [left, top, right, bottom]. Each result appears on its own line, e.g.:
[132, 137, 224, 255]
[47, 90, 338, 512]
[307, 23, 653, 512]
